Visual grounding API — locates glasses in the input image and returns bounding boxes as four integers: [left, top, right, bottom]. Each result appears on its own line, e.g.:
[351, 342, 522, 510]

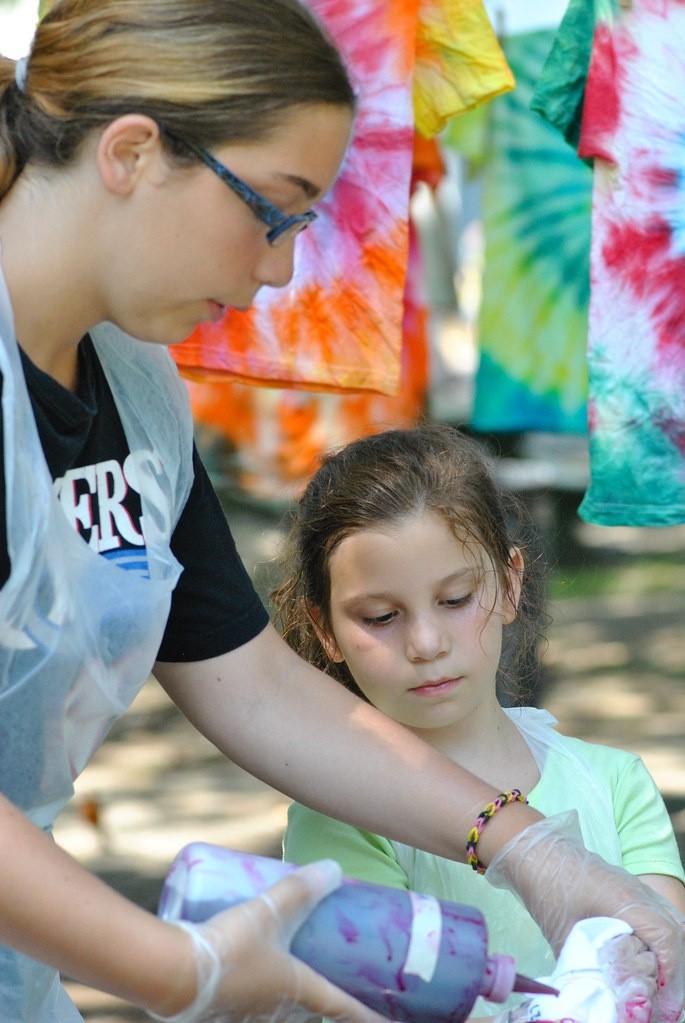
[160, 124, 318, 248]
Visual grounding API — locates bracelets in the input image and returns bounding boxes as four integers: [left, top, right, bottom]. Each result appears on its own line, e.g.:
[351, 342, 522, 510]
[466, 788, 528, 876]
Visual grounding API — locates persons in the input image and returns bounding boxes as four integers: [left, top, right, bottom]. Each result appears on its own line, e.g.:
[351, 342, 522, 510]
[266, 423, 685, 1023]
[0, 0, 685, 1023]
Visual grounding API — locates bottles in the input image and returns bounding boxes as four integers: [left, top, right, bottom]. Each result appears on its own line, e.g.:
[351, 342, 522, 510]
[158, 842, 561, 1023]
